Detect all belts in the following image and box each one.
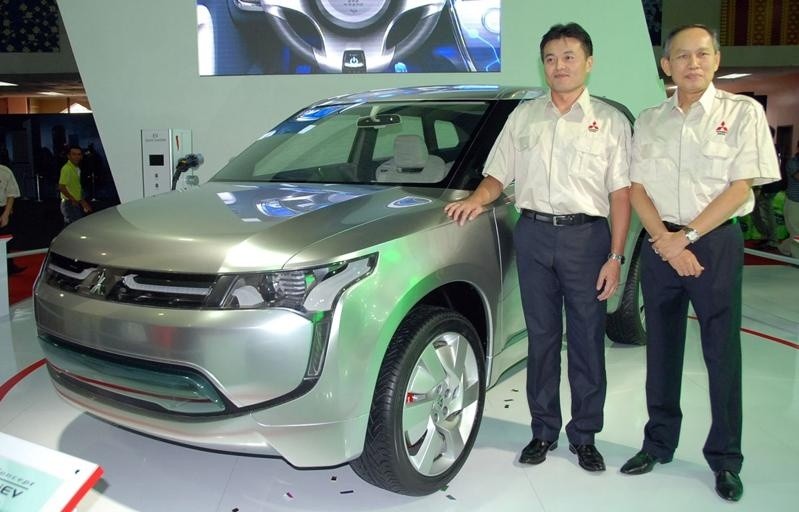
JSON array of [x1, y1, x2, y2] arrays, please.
[[663, 217, 737, 232], [521, 208, 602, 226]]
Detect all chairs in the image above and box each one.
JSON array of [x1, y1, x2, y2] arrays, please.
[[374, 134, 446, 184]]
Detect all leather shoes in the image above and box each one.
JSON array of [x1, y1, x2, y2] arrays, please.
[[569, 440, 605, 471], [620, 448, 673, 475], [519, 437, 558, 463], [715, 469, 743, 502]]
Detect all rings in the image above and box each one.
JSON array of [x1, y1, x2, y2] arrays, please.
[[654, 248, 660, 255]]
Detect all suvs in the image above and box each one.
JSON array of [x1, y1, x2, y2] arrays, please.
[[32, 84, 647, 498]]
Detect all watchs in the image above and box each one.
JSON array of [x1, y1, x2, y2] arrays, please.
[[684, 224, 701, 245], [606, 252, 627, 266]]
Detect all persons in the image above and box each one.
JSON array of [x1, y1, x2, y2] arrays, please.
[[56, 146, 92, 222], [1, 164, 29, 276], [443, 21, 633, 471], [619, 23, 782, 500], [739, 125, 798, 258]]
